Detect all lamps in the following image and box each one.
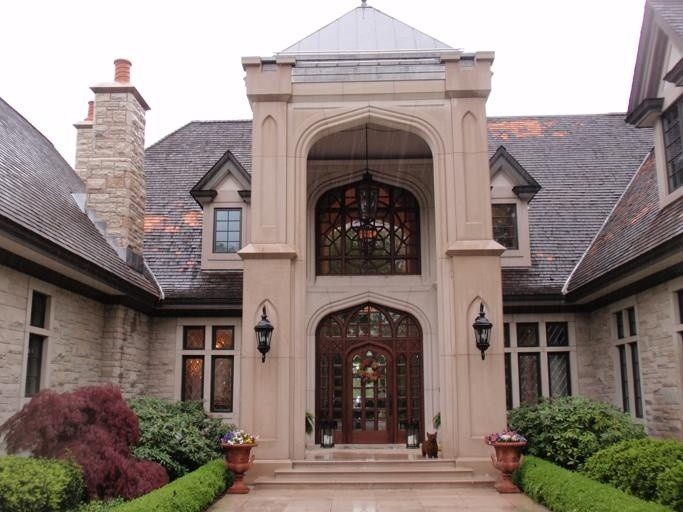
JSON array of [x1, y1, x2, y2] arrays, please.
[[254, 305, 274, 363], [472, 301, 492, 360], [355, 123, 380, 222]]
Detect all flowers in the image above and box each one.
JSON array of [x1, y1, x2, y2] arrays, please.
[[487, 430, 527, 442], [223, 429, 255, 444]]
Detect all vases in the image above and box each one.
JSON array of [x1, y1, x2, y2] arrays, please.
[[222, 444, 257, 494], [491, 442, 527, 493]]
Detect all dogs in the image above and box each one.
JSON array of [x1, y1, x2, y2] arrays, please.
[[421, 431, 439, 459]]
